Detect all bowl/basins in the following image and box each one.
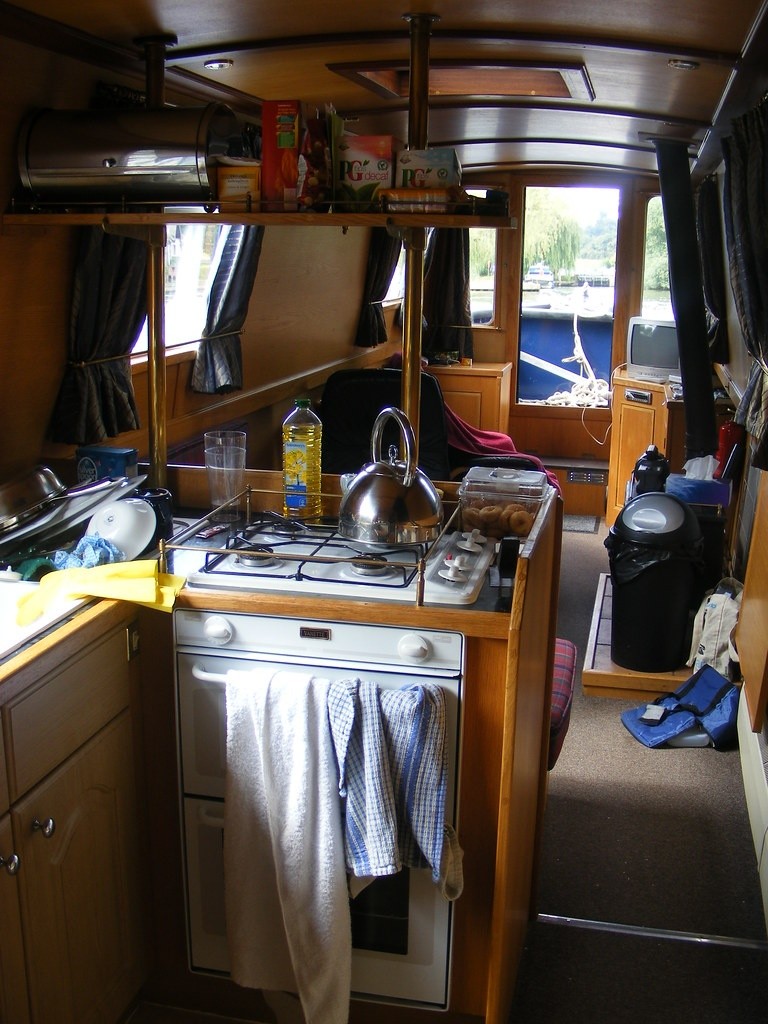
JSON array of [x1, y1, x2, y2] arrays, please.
[[436, 488, 444, 500]]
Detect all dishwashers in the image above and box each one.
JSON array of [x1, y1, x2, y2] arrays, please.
[[175, 609, 467, 1013]]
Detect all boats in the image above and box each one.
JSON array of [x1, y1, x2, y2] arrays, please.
[[522, 261, 554, 292]]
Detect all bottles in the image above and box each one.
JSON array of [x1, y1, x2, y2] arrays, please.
[[283, 398, 322, 525]]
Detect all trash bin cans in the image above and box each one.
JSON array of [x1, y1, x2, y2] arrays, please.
[[602, 491, 705, 672]]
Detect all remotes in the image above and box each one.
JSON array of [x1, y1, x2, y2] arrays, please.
[[635, 376, 665, 383]]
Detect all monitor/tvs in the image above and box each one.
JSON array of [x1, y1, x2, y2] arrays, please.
[[626, 317, 681, 380]]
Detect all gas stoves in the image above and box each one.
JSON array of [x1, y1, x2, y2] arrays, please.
[[187, 519, 500, 604]]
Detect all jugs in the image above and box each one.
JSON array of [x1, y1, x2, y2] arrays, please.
[[338, 407, 444, 544]]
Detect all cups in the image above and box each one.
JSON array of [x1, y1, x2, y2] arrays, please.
[[135, 488, 173, 542], [340, 474, 357, 494], [203, 430, 246, 522]]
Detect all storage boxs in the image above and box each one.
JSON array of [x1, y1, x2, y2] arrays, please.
[[332, 135, 461, 211], [456, 466, 551, 541], [217, 167, 262, 214], [76, 446, 139, 483]]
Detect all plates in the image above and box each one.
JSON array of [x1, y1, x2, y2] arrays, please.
[[85, 498, 156, 561]]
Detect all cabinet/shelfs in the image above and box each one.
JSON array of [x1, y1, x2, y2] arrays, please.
[[0, 598, 185, 1024]]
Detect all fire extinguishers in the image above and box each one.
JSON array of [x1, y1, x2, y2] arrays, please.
[[713, 407, 744, 480]]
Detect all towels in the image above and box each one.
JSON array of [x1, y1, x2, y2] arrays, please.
[[328, 677, 464, 901], [226, 670, 350, 1024]]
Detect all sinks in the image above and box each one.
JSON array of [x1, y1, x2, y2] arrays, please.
[[0, 581, 97, 662]]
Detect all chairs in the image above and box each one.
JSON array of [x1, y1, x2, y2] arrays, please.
[[322, 367, 537, 483]]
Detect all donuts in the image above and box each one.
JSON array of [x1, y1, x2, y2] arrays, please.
[[462, 499, 533, 535]]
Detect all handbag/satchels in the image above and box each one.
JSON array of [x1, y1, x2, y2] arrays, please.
[[620, 664, 740, 749]]
